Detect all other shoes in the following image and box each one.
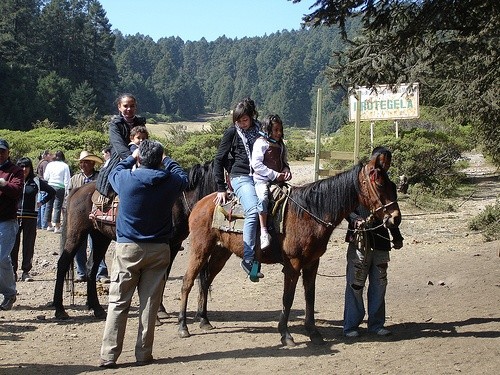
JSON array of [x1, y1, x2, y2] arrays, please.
[[47, 225, 62, 234], [346, 331, 359, 337], [100, 278, 110, 283], [242, 259, 264, 278], [73, 277, 86, 283], [38, 226, 47, 230], [137, 357, 154, 366], [376, 328, 392, 336], [97, 359, 117, 368]]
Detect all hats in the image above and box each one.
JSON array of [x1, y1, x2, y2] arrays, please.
[[0, 139, 9, 150], [72, 151, 103, 164]]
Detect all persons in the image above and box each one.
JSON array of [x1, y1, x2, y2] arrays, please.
[[343, 147, 393, 339], [127, 128, 155, 172], [98, 96, 145, 197], [251, 114, 291, 248], [0, 141, 110, 306], [98, 143, 189, 367], [213, 100, 268, 278]]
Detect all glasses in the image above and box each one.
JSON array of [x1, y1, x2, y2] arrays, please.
[[20, 165, 31, 168]]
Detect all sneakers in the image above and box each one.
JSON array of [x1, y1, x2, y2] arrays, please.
[[22, 273, 33, 282], [260, 233, 272, 249], [0, 295, 16, 310]]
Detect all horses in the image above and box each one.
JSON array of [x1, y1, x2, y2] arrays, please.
[[177, 152, 404, 348], [50, 156, 236, 320]]
